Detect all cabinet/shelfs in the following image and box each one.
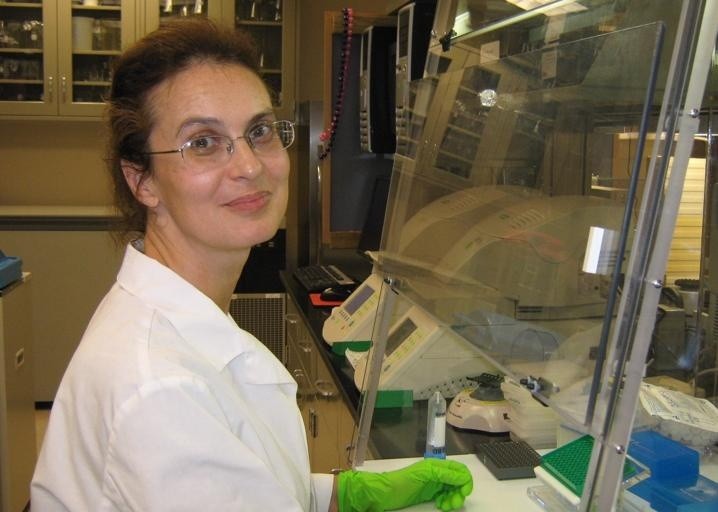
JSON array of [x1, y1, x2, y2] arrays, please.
[[283, 290, 374, 473], [141, 0, 295, 121], [0, 0, 141, 123]]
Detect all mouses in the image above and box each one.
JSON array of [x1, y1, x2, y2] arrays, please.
[[320, 287, 352, 301]]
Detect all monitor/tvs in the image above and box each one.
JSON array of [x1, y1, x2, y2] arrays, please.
[[357, 176, 391, 265]]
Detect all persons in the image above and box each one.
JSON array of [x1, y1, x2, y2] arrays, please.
[[30, 14, 473, 512]]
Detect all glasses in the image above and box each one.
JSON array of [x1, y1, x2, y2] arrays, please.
[[133, 118, 297, 170]]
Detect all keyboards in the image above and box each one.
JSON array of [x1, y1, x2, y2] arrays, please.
[[293, 264, 355, 292]]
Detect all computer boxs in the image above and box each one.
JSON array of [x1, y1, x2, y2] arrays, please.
[[360, 25, 396, 153], [396, 0, 438, 147]]
